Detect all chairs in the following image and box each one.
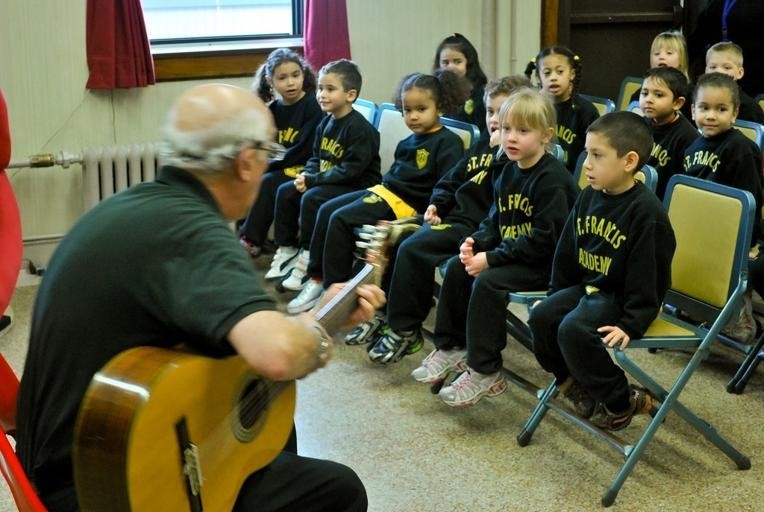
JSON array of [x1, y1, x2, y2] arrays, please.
[[0, 355, 53, 512], [734, 120, 760, 144], [352, 98, 659, 444], [517, 174, 760, 506], [575, 77, 646, 117]]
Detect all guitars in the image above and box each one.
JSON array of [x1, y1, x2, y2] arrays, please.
[[74, 225, 390, 511]]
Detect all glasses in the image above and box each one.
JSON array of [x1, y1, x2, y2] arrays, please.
[[258, 143, 286, 160]]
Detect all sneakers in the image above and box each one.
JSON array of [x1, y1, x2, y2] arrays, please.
[[345, 313, 423, 367], [264, 247, 324, 314], [411, 350, 507, 408], [560, 377, 654, 431], [722, 296, 757, 343]]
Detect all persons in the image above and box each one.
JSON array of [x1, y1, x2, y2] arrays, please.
[[411, 84, 582, 409], [527, 111, 676, 431], [525, 45, 600, 178], [234, 47, 328, 257], [433, 32, 488, 155], [286, 67, 473, 318], [263, 59, 382, 293], [15, 80, 368, 510], [344, 74, 534, 365]]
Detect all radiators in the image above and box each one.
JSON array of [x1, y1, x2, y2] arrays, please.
[[83, 139, 163, 215]]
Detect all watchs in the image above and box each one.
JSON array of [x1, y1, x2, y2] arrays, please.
[[313, 325, 331, 369]]
